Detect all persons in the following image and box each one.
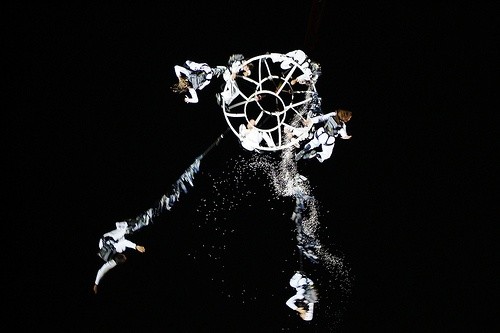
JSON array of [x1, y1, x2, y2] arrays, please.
[[236, 120, 268, 153], [297, 106, 356, 164], [267, 49, 323, 89], [286, 273, 319, 321], [213, 53, 254, 111], [90, 218, 147, 294], [172, 60, 226, 104]]
